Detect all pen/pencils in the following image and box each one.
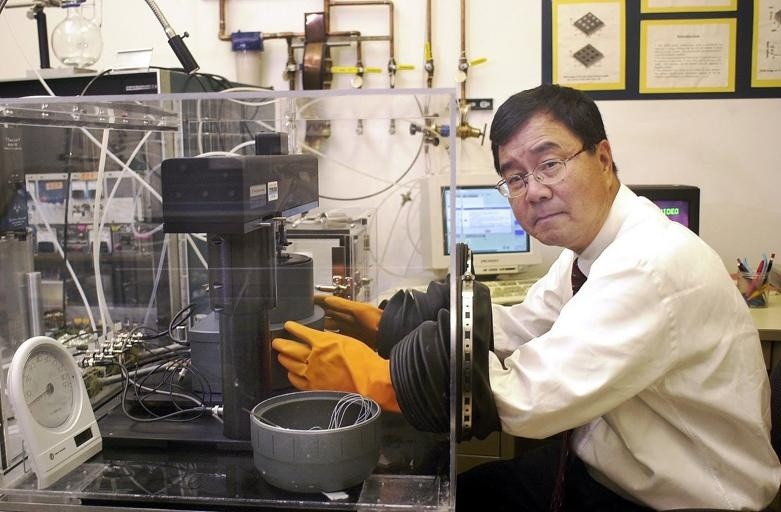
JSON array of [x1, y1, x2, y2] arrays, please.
[[736, 253, 775, 307]]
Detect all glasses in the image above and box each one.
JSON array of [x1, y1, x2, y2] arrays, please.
[[494, 143, 594, 199]]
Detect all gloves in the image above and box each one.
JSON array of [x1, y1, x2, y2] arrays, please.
[[271, 320, 404, 416], [313, 294, 384, 345]]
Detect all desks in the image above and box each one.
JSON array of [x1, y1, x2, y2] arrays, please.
[[457, 287, 781, 479]]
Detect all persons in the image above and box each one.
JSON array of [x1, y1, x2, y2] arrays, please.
[[272, 85, 781, 511]]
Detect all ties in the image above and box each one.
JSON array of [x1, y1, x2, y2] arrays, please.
[[571, 256, 587, 296]]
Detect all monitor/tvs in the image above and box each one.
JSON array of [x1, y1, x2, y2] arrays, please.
[[625, 184, 700, 237], [429, 174, 542, 274]]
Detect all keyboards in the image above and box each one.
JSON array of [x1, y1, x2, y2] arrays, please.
[[480, 279, 539, 306]]
